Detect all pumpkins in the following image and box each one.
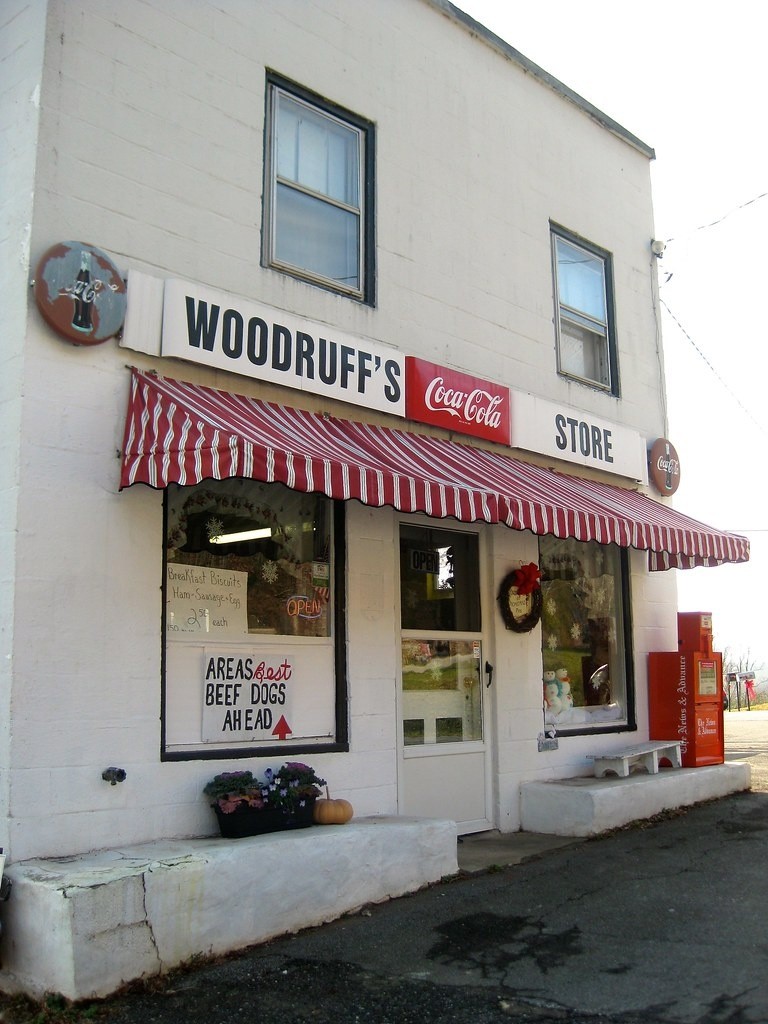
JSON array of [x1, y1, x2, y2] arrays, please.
[[313, 786, 353, 824]]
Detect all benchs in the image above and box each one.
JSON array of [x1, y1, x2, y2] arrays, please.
[[585, 739, 685, 777]]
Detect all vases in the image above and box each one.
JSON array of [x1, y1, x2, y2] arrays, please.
[[207, 794, 320, 839]]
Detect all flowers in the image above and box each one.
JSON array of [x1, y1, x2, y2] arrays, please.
[[200, 770, 264, 814], [262, 760, 328, 814]]
